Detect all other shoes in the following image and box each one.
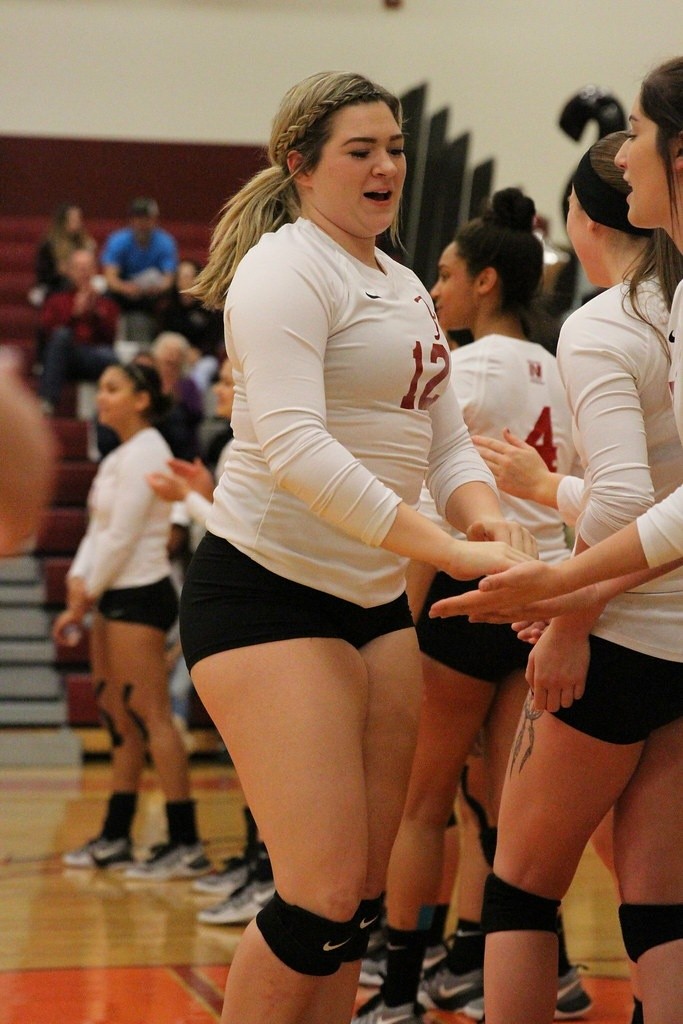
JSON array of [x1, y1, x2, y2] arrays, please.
[[353, 922, 591, 1023], [64, 832, 274, 923]]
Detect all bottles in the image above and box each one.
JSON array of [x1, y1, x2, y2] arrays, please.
[[53, 617, 82, 646]]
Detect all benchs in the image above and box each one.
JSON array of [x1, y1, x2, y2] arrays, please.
[[1, 214, 229, 729]]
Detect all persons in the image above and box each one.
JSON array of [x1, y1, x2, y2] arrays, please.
[[172, 70, 539, 1024], [351, 57, 683, 1024], [1, 200, 276, 923]]
